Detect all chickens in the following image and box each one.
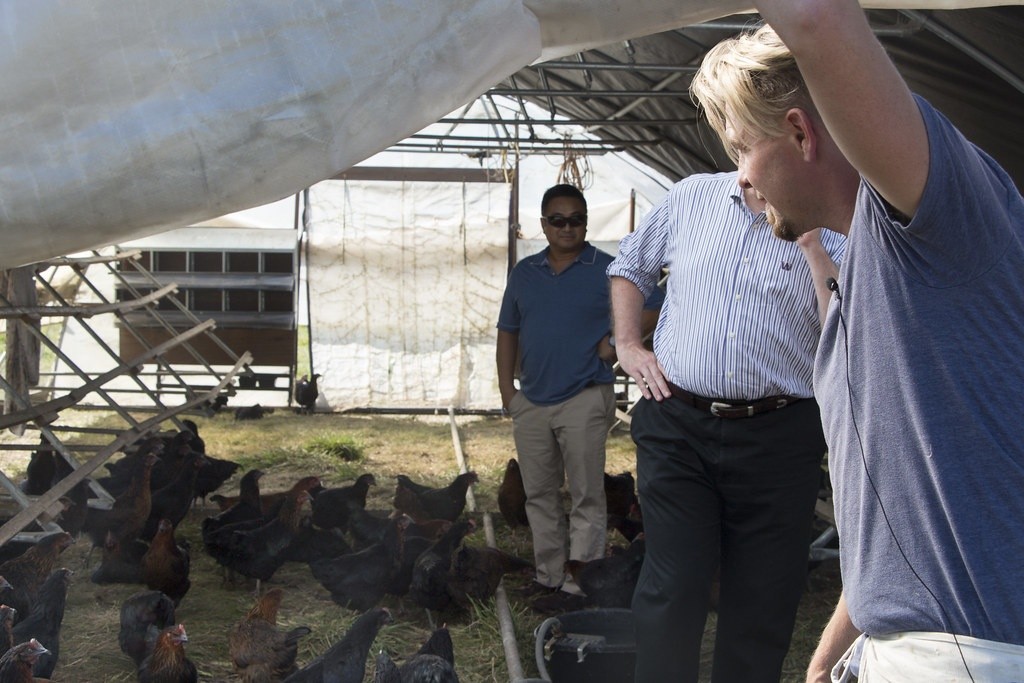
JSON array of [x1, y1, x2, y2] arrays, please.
[[607, 509, 642, 541], [118, 589, 176, 669], [136, 622, 198, 683], [278, 606, 395, 683], [396, 622, 459, 683], [18, 418, 549, 629], [561, 549, 642, 608], [615, 392, 629, 421], [0, 497, 78, 566], [604, 471, 638, 517], [0, 531, 78, 683], [374, 648, 402, 683], [227, 587, 312, 683], [295, 373, 322, 415]]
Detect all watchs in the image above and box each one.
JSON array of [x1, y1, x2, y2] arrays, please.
[[607, 332, 615, 349]]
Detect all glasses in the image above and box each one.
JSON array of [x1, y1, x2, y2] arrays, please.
[[543, 214, 587, 228]]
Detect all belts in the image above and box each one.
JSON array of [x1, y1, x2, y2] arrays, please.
[[664, 381, 800, 419]]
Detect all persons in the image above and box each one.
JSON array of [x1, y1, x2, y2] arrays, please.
[[688, 1, 1023, 683], [604, 102, 850, 683], [496, 184, 668, 618]]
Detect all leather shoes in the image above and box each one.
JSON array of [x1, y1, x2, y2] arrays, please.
[[513, 581, 586, 615]]
